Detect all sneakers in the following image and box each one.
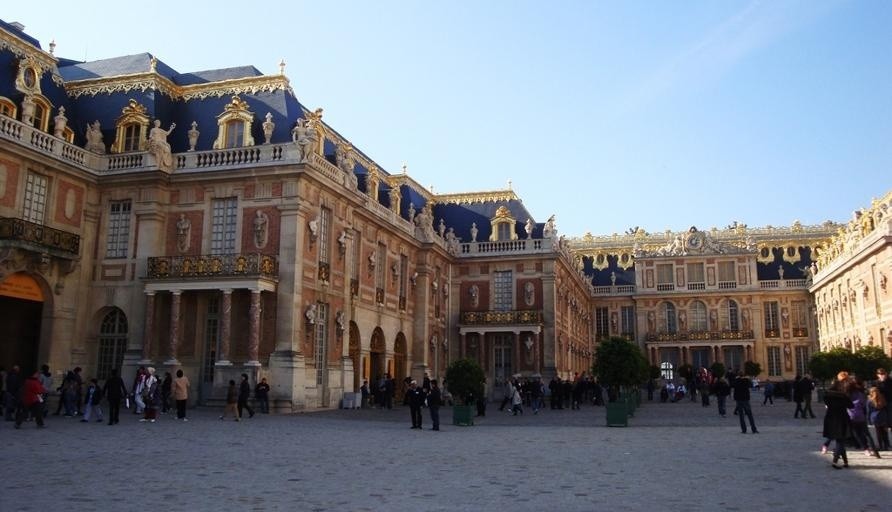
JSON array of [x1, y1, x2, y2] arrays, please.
[[832, 464, 850, 470], [740, 431, 746, 434], [821, 445, 828, 455], [864, 450, 873, 457], [752, 430, 760, 434], [248, 411, 255, 418], [138, 418, 156, 423]]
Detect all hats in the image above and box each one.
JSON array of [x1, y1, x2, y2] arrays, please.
[[147, 367, 156, 376]]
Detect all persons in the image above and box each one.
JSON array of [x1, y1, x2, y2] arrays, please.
[[220, 380, 240, 422], [0, 363, 190, 430], [292, 118, 316, 164], [237, 373, 255, 419], [256, 377, 270, 414], [657, 365, 775, 434], [800, 371, 816, 419], [793, 375, 806, 419], [414, 208, 432, 239], [819, 367, 892, 470], [360, 373, 454, 431], [497, 370, 606, 417], [147, 120, 176, 167]]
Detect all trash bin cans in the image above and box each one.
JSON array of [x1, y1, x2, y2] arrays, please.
[[343, 392, 362, 410]]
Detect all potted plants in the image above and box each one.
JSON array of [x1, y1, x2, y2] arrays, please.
[[444, 359, 487, 427], [809, 348, 831, 404], [593, 333, 648, 425]]
